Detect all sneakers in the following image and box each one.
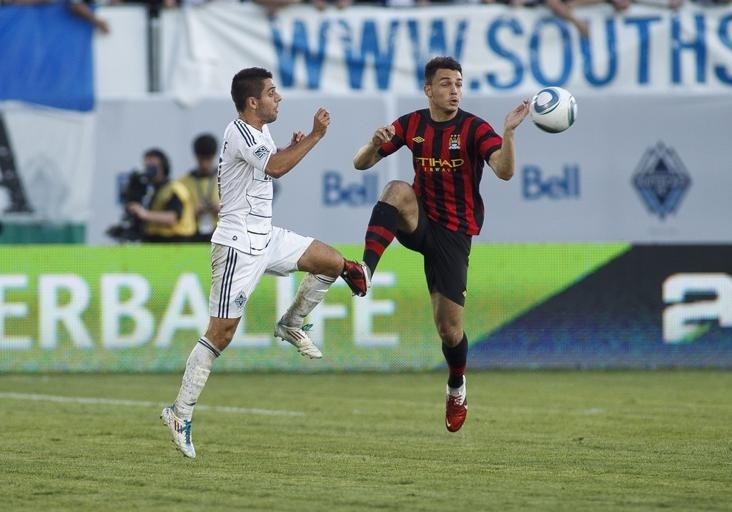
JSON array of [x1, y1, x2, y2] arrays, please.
[[445, 375, 468, 432], [340, 257, 371, 297], [273, 320, 323, 358], [160, 406, 197, 458]]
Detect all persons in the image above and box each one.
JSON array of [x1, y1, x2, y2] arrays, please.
[[160, 66, 349, 460], [123, 148, 199, 241], [179, 134, 220, 242], [338, 57, 532, 432], [67, 0, 686, 41]]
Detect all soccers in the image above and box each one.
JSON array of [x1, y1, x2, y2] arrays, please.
[[531, 87, 576, 133]]
